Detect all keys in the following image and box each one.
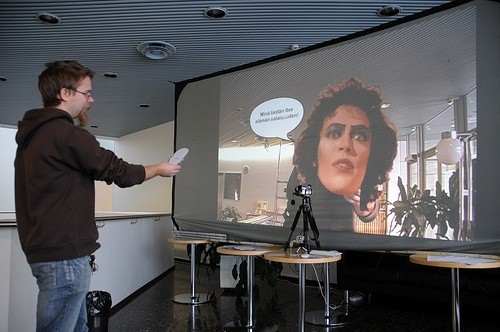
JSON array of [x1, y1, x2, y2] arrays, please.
[[88, 254, 98, 273]]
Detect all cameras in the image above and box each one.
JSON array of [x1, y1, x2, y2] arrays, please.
[[292, 185, 313, 196]]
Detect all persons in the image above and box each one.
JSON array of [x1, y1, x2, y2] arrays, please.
[[282, 78, 398, 231], [14, 60, 181, 332]]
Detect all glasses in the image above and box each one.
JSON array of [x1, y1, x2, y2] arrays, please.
[[65, 87, 92, 97]]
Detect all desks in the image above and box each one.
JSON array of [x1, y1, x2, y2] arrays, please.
[[167, 238, 354, 332], [410, 254, 500, 332]]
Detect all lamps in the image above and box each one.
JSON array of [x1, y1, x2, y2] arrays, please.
[[435, 132, 478, 166]]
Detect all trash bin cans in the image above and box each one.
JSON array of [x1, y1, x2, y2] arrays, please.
[[85, 288, 113, 330]]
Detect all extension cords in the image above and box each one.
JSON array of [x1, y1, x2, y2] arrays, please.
[[350, 296, 363, 302]]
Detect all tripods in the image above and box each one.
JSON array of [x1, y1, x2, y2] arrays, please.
[[284, 196, 321, 254]]
